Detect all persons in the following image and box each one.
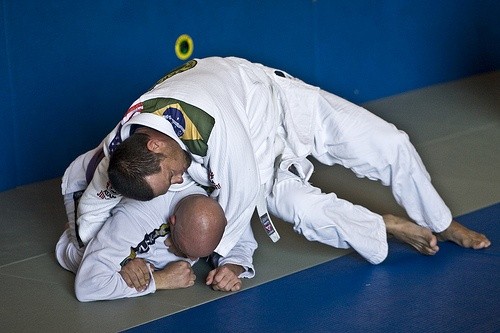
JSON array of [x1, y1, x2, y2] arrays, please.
[[75, 55, 492, 292], [54, 143, 260, 303]]
